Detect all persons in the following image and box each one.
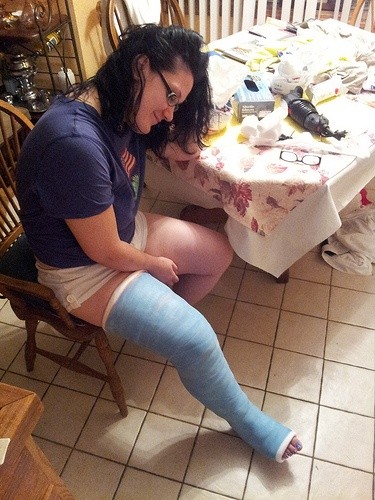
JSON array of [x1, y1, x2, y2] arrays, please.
[[16, 23, 302, 464]]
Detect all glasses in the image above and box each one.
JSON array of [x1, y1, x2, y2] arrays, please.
[[279, 130, 322, 166], [150, 62, 179, 112]]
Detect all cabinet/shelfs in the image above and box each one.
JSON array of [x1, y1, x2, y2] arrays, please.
[[0, 0, 85, 125]]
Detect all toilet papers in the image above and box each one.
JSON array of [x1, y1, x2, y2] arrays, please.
[[256, 98, 289, 134], [262, 120, 287, 143], [240, 115, 259, 137]]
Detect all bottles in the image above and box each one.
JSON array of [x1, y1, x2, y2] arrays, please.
[[21, 0, 43, 31], [58, 67, 79, 98], [31, 28, 64, 61]]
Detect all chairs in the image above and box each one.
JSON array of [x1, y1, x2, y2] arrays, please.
[[0, 99, 131, 417], [106, 0, 186, 52]]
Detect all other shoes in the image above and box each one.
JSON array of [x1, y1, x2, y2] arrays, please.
[[179, 204, 228, 223]]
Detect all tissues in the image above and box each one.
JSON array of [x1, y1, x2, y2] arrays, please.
[[271, 59, 310, 96], [230, 74, 275, 123]]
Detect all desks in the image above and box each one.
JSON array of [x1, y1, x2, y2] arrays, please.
[[146, 16, 375, 283], [0, 383, 76, 500]]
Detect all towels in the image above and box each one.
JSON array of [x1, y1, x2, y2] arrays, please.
[[320, 202, 375, 276], [108, 0, 162, 39]]
